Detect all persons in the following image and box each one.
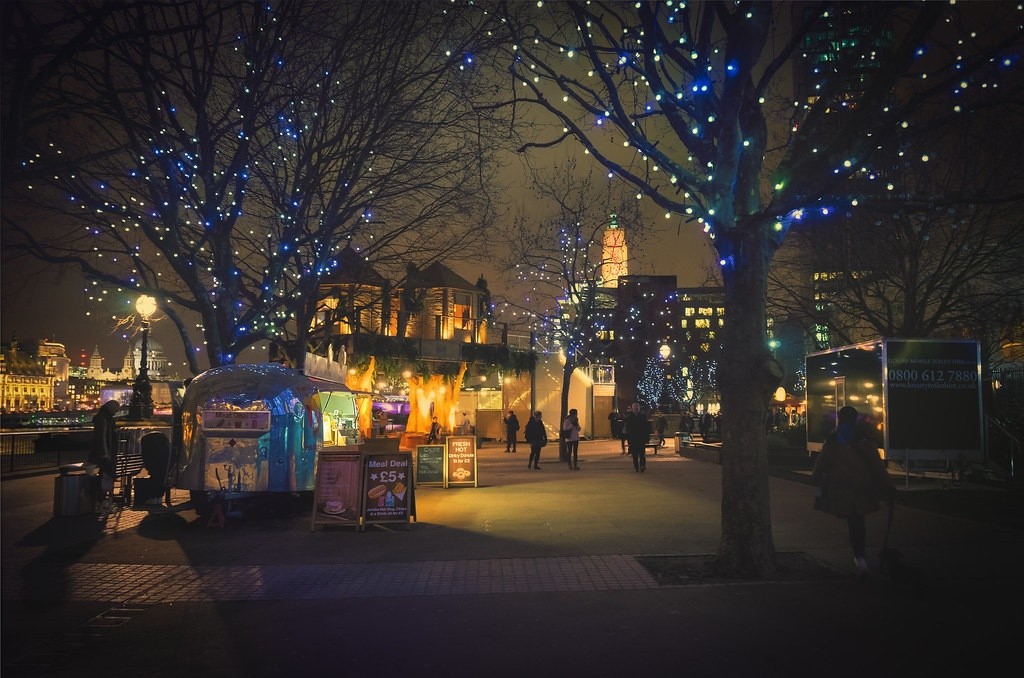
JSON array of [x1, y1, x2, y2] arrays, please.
[[716, 411, 721, 435], [810, 406, 896, 580], [428, 417, 439, 444], [621, 403, 650, 473], [679, 412, 694, 439], [503, 411, 519, 453], [698, 409, 711, 438], [655, 413, 668, 446], [563, 409, 581, 470], [524, 411, 547, 470], [456, 413, 470, 436], [608, 408, 618, 440], [89, 400, 120, 514]]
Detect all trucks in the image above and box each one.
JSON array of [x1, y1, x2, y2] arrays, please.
[[169, 363, 386, 512]]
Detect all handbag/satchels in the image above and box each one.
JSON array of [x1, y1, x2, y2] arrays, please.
[[560, 430, 571, 438]]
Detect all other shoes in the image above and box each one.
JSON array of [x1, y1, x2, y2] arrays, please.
[[512, 449, 516, 452], [640, 467, 645, 472], [855, 558, 867, 569], [534, 464, 541, 469], [504, 450, 510, 453], [574, 466, 580, 471], [635, 466, 639, 471], [568, 465, 573, 472], [528, 465, 531, 471]]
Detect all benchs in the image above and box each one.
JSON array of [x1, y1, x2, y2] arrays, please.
[[59, 453, 144, 503], [623, 433, 660, 455]]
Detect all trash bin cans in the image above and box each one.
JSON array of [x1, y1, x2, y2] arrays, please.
[[674, 432, 690, 453], [53, 462, 100, 522]]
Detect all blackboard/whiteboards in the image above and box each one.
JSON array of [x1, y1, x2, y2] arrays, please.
[[446, 436, 478, 484], [361, 451, 417, 524], [415, 444, 446, 484], [310, 451, 364, 525]]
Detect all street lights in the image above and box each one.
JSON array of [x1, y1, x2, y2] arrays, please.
[[129, 294, 156, 418]]
